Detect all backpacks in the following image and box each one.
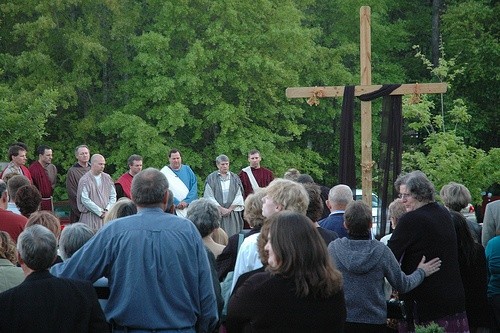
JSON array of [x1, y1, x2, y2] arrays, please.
[[481, 181, 500, 219]]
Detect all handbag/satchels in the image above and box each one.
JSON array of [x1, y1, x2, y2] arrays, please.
[[219, 233, 244, 317]]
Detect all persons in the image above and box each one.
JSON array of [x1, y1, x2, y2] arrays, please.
[[76, 154, 117, 230], [204, 155, 246, 240], [66, 145, 93, 218], [49, 167, 219, 333], [238, 150, 274, 198], [114, 154, 144, 200], [0, 143, 33, 184], [0, 167, 500, 333], [160, 149, 197, 219], [29, 145, 57, 211]]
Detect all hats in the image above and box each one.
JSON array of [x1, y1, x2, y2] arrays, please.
[[297, 174, 314, 184]]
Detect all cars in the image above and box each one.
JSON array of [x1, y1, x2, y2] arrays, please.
[[53, 200, 71, 231], [352, 188, 390, 237]]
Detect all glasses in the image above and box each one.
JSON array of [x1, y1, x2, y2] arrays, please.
[[397, 193, 409, 198]]
